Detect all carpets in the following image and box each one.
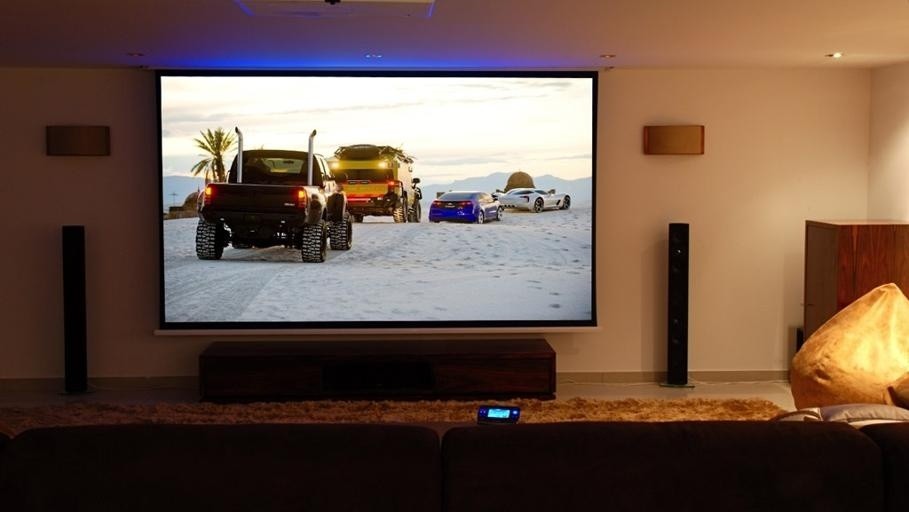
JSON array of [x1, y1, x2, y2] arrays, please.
[[0, 398, 797, 438]]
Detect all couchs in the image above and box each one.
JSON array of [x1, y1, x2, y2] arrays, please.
[[0, 420, 909, 512]]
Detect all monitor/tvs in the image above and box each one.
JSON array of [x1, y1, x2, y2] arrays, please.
[[153, 69, 602, 338]]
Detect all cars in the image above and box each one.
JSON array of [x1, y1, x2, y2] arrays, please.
[[491, 187, 571, 213]]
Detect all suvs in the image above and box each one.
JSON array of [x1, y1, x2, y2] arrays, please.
[[324, 144, 422, 223], [195, 126, 353, 263]]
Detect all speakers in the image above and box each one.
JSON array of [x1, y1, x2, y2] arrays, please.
[[57, 225, 97, 394], [659, 222, 695, 388]]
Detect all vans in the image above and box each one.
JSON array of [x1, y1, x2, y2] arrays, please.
[[429, 191, 504, 224]]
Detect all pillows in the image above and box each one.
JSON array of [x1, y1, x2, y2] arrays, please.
[[791, 283, 909, 410]]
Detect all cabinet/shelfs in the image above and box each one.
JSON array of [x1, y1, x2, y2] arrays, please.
[[803, 218, 909, 343], [197, 340, 556, 403]]
